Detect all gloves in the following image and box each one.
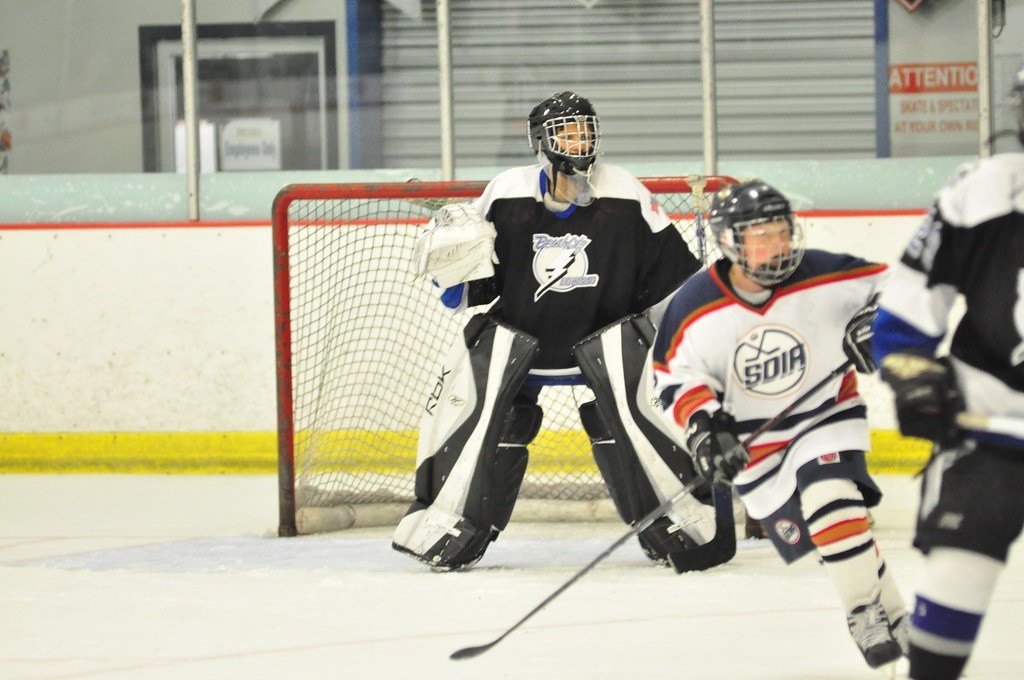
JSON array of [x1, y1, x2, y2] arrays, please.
[[843, 293, 882, 373], [883, 358, 971, 448], [685, 407, 751, 480]]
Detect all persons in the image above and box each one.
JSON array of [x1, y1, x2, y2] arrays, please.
[[644, 179, 910, 669], [872, 70, 1024, 680], [386, 93, 736, 574]]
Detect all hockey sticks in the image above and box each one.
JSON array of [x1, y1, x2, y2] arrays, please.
[[448, 355, 856, 662]]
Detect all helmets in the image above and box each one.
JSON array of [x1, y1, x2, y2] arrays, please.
[[710, 180, 806, 286], [527, 92, 601, 176]]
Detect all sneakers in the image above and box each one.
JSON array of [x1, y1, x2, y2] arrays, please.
[[847, 591, 911, 667]]
